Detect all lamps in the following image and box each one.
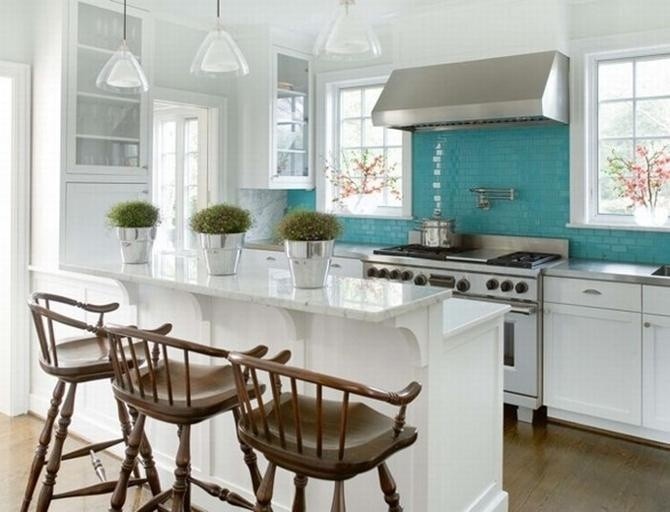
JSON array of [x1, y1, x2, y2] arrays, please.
[[311, 0, 382, 66], [192, 1, 250, 80], [94, 0, 150, 97]]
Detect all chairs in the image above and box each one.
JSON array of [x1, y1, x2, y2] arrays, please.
[[98, 323, 266, 511], [223, 352, 422, 511], [20, 293, 162, 511]]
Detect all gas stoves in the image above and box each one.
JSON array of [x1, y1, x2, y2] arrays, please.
[[373, 243, 561, 269]]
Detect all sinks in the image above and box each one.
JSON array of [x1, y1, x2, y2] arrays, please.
[[650, 265, 670, 278]]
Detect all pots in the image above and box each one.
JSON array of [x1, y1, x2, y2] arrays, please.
[[407, 208, 456, 249]]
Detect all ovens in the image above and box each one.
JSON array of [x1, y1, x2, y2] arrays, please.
[[492, 295, 545, 411]]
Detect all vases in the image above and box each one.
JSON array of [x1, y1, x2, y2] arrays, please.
[[344, 194, 377, 213], [634, 206, 668, 226]]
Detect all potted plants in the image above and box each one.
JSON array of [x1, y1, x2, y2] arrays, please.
[[189, 203, 254, 277], [103, 201, 162, 264], [276, 212, 341, 289]]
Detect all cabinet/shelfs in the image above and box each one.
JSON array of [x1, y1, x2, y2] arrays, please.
[[642, 282, 670, 445], [240, 40, 315, 192], [32, 0, 152, 267], [543, 269, 642, 439], [244, 245, 362, 280]]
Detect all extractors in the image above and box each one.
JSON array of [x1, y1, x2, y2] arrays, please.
[[371, 51, 570, 132]]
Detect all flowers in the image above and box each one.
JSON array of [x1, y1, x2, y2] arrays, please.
[[319, 146, 402, 205], [605, 139, 670, 210]]
